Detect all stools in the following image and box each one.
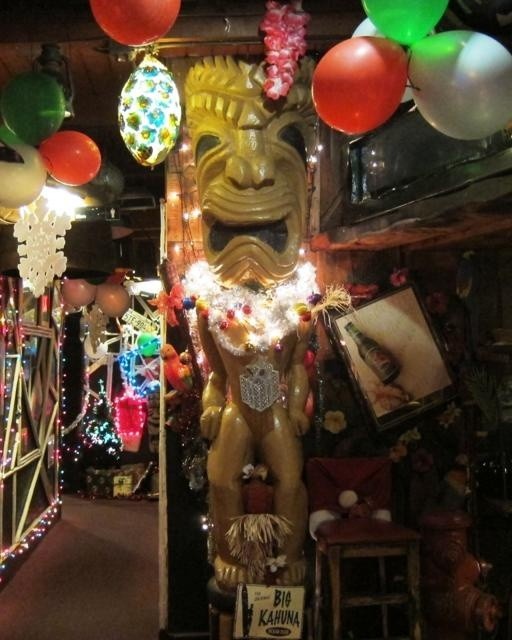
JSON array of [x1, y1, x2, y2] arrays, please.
[[314, 516, 425, 636]]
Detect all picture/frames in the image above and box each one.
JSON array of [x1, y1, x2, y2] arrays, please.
[[332, 280, 464, 434]]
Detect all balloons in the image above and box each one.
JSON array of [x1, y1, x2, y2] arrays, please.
[[62, 278, 131, 318], [0, 143, 47, 207], [90, 0, 181, 46], [137, 334, 160, 358], [0, 71, 65, 147], [38, 131, 102, 186], [311, 0, 512, 140]]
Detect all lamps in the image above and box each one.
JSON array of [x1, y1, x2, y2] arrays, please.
[[116, 53, 182, 169]]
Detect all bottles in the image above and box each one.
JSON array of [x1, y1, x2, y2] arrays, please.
[[342, 321, 402, 385]]
